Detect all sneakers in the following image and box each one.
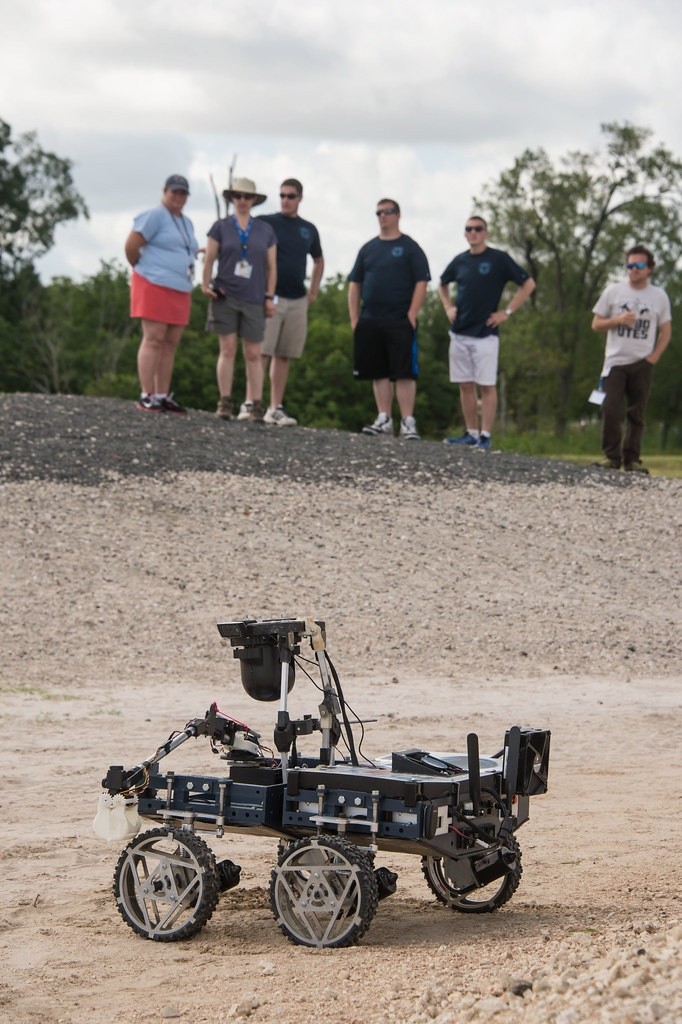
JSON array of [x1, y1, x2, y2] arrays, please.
[[135, 395, 165, 413], [399, 416, 420, 439], [362, 413, 393, 435], [473, 435, 491, 452], [444, 432, 479, 446], [153, 393, 187, 415], [262, 408, 297, 427], [237, 405, 263, 420]]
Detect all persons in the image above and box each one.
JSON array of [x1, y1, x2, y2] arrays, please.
[[124, 175, 197, 414], [348, 199, 432, 439], [195, 176, 324, 428], [591, 245, 672, 474], [436, 216, 537, 449]]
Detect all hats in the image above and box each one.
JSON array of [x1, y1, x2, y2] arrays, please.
[[222, 178, 266, 206], [166, 175, 189, 194]]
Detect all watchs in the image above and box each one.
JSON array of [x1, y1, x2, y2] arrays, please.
[[505, 308, 512, 315]]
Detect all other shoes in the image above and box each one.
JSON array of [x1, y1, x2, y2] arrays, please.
[[592, 459, 620, 469], [216, 401, 234, 419], [625, 459, 649, 474]]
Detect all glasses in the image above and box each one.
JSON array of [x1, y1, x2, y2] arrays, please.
[[466, 226, 486, 232], [233, 193, 251, 200], [280, 192, 300, 199], [627, 262, 649, 269], [377, 208, 397, 215]]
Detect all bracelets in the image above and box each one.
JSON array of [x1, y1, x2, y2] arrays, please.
[[265, 292, 275, 299]]
